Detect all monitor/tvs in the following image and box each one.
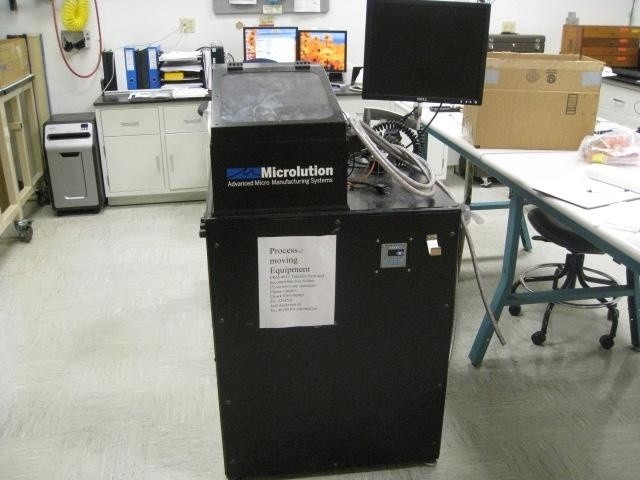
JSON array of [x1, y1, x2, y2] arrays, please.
[[242, 26, 298, 70], [299, 30, 349, 89], [361, 0, 491, 106]]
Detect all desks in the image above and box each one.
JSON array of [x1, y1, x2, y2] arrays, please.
[[393, 94, 639, 368]]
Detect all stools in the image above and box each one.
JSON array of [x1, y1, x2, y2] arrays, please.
[[509, 203, 622, 353]]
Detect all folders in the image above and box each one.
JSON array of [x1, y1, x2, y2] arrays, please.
[[147, 44, 161, 89], [124, 48, 138, 90]]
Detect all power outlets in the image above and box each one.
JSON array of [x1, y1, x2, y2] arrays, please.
[[180, 17, 195, 32]]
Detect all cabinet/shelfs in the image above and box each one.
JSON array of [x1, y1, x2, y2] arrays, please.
[[98, 102, 211, 197], [599, 81, 639, 130], [333, 93, 449, 179]]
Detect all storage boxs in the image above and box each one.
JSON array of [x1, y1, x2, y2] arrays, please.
[[461, 47, 602, 153]]
